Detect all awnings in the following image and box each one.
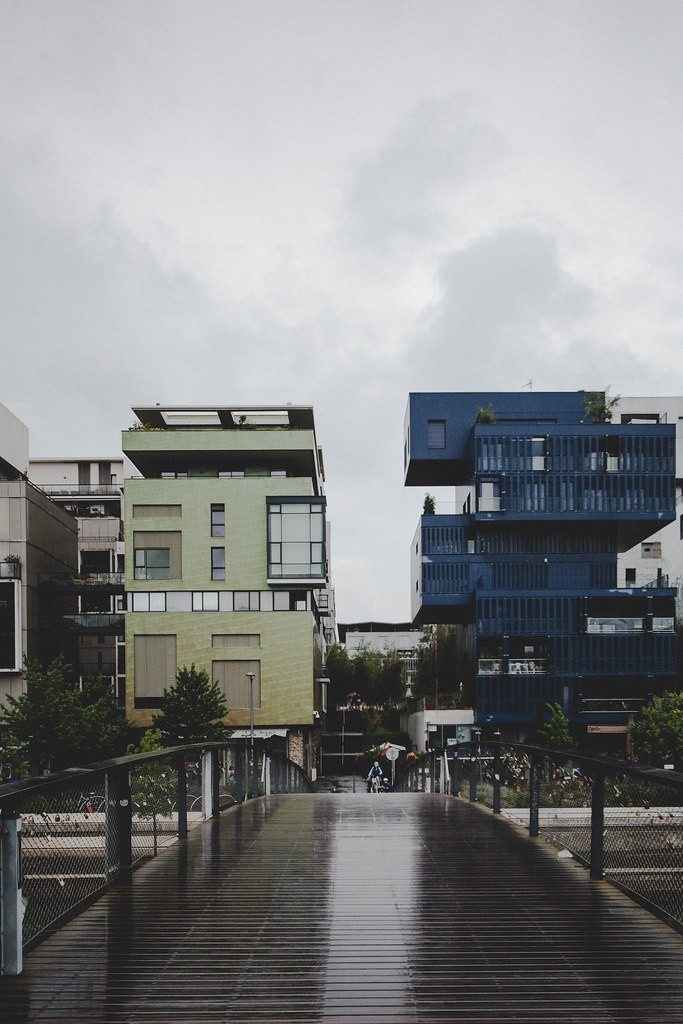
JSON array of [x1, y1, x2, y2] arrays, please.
[[157, 728, 290, 740]]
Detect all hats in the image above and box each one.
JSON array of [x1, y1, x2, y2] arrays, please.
[[374, 762, 379, 767], [383, 778, 388, 782]]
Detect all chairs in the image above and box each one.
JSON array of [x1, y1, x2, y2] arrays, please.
[[492, 662, 539, 673]]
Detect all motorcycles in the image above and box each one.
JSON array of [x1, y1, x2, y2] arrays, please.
[[367, 773, 390, 793]]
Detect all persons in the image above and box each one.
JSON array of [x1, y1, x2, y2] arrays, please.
[[227, 766, 236, 787], [366, 761, 383, 787], [492, 660, 537, 674], [381, 777, 392, 793]]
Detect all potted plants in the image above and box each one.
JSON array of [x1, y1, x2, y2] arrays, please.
[[4, 555, 20, 576]]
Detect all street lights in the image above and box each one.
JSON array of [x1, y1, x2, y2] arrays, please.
[[246, 669, 256, 763]]
